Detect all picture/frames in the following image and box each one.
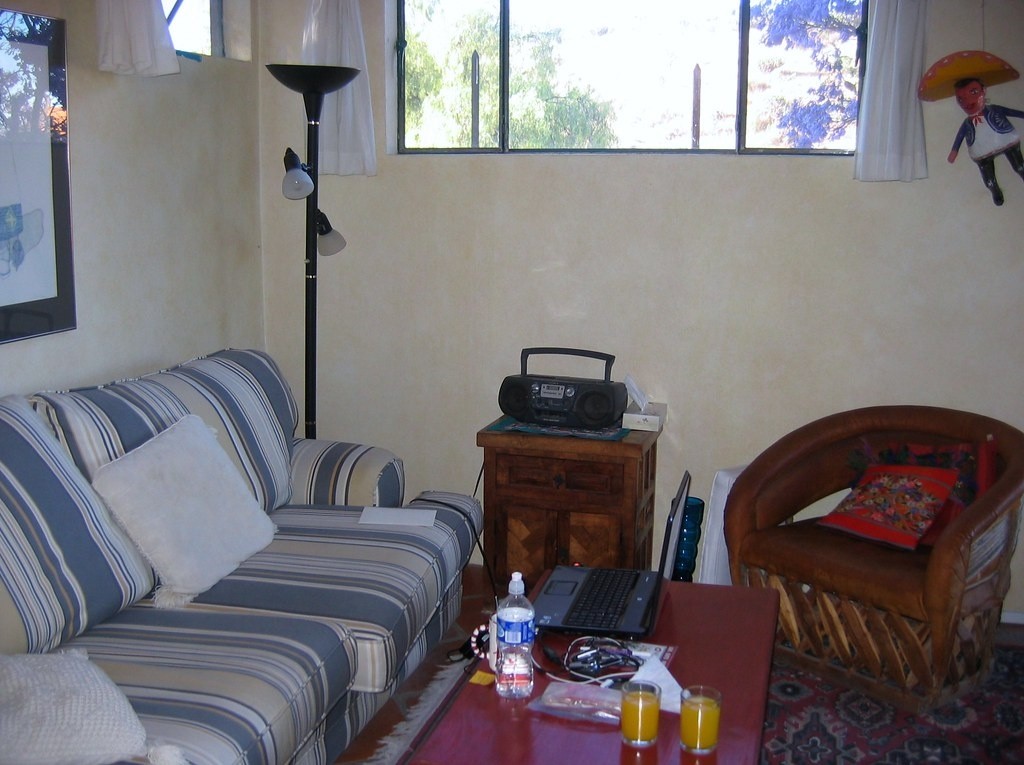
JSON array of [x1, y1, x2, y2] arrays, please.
[[0, 9, 78, 344]]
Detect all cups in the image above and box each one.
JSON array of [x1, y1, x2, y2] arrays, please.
[[679, 684, 723, 755], [620, 680, 662, 748], [489, 608, 533, 674]]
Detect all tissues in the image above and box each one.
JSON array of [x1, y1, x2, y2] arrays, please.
[[622, 374, 668, 432]]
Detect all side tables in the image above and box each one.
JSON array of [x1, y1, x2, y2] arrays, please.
[[478, 402, 663, 598]]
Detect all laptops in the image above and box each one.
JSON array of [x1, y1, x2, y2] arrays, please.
[[531, 471, 691, 639]]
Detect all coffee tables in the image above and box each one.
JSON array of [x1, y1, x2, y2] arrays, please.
[[404, 568, 780, 765]]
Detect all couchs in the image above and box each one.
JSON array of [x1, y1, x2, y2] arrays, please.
[[0, 346, 484, 765], [722, 405, 1024, 714]]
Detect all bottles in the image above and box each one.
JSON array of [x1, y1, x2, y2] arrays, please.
[[496, 571, 535, 698]]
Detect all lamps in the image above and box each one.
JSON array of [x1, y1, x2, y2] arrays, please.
[[282, 147, 315, 201], [317, 208, 346, 257]]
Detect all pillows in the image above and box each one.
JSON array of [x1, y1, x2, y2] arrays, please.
[[91, 415, 280, 608], [815, 439, 998, 550], [0, 647, 192, 765]]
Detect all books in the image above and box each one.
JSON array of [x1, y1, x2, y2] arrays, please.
[[358, 506, 437, 526]]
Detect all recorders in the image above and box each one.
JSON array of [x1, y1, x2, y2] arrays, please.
[[498, 348, 629, 431]]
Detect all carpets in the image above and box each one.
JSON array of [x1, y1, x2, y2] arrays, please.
[[360, 629, 1023, 765]]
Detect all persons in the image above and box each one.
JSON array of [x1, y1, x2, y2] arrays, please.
[[947, 77, 1024, 206]]
